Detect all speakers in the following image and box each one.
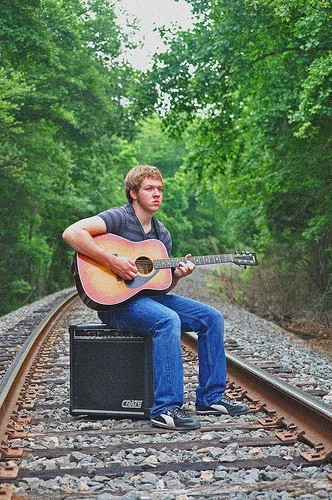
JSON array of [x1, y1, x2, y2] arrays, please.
[[68, 322, 155, 420]]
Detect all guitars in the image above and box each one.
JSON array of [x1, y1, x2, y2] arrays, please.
[[71, 231, 259, 311]]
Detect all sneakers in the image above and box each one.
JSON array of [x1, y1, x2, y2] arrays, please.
[[194, 395, 252, 416], [148, 408, 201, 430]]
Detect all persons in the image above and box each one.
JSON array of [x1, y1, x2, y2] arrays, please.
[[63, 164, 249, 430]]
